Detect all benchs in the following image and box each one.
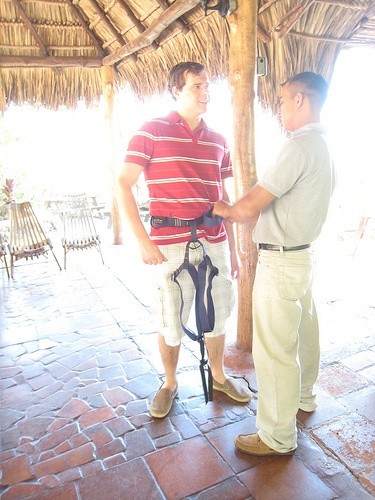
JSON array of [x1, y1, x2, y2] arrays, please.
[[54, 206, 105, 221]]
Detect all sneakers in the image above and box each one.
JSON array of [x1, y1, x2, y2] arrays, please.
[[149, 381, 179, 418], [234, 433, 296, 456], [212, 376, 253, 403]]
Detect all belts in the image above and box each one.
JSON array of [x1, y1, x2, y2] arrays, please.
[[258, 243, 312, 251]]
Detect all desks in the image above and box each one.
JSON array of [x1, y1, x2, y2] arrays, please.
[[44, 195, 104, 221]]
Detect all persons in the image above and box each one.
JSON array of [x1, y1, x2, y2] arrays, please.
[[114, 63, 252, 418], [212, 72, 335, 455]]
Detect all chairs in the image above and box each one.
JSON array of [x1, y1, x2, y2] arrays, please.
[[0, 192, 105, 278]]
